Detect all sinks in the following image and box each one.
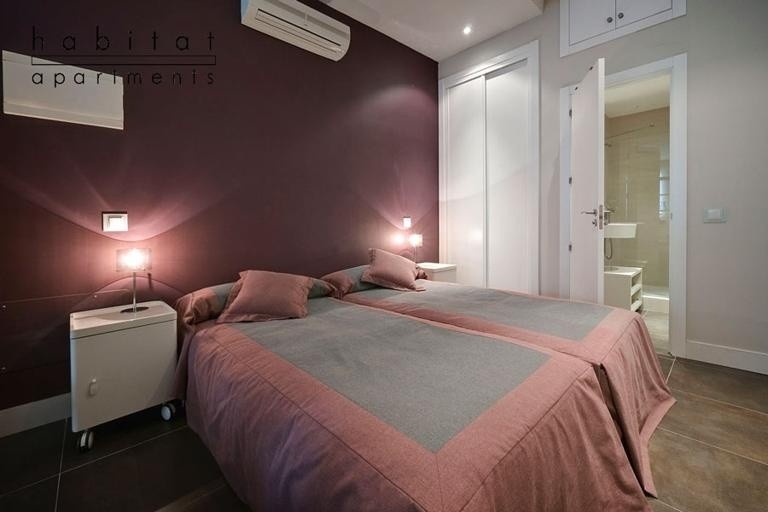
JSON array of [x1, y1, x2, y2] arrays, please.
[[603, 222, 645, 239]]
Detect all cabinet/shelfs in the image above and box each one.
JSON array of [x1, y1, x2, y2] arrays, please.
[[603, 262, 644, 314], [434, 40, 541, 300], [558, 0, 688, 57]]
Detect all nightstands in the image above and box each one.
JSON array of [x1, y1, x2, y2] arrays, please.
[[414, 261, 457, 284], [64, 299, 181, 452]]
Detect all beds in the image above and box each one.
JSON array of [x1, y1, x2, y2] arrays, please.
[[169, 273, 650, 512], [318, 261, 678, 502]]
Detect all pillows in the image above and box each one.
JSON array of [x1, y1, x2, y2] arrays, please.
[[360, 246, 428, 293], [172, 274, 336, 325], [216, 267, 311, 324], [322, 263, 428, 297]]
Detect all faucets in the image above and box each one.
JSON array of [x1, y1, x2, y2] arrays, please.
[[605, 210, 613, 225]]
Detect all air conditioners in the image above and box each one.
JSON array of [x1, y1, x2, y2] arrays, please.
[[238, 0, 351, 62]]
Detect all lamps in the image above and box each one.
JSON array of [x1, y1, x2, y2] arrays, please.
[[409, 231, 423, 264], [115, 245, 151, 316]]
